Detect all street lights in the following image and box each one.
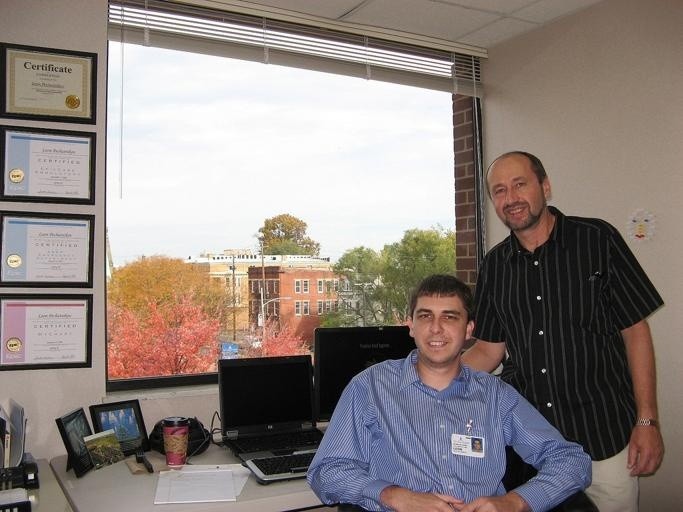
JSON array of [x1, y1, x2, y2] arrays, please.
[[260, 286, 293, 357]]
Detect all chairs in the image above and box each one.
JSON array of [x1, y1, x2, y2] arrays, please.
[[498, 441, 600, 511]]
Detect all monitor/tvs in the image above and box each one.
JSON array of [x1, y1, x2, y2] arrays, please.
[[313, 325, 417, 421]]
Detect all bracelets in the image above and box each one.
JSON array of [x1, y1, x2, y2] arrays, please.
[[634, 417, 660, 428]]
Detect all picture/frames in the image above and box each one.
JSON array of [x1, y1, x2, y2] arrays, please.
[[1, 123, 98, 207], [55, 407, 94, 480], [0, 291, 95, 372], [0, 209, 96, 291], [88, 397, 155, 456], [0, 42, 99, 125]]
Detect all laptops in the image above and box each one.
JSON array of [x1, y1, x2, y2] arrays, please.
[[217, 354, 324, 462]]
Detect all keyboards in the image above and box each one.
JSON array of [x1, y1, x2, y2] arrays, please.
[[246, 453, 317, 482]]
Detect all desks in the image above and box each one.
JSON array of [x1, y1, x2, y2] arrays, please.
[[49, 438, 342, 511], [0, 454, 76, 512]]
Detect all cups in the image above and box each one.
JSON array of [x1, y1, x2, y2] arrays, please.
[[160, 416, 190, 466]]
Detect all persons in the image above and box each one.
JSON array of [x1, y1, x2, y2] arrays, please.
[[307, 273, 591, 512], [472, 437, 483, 453], [458, 152, 663, 512]]
